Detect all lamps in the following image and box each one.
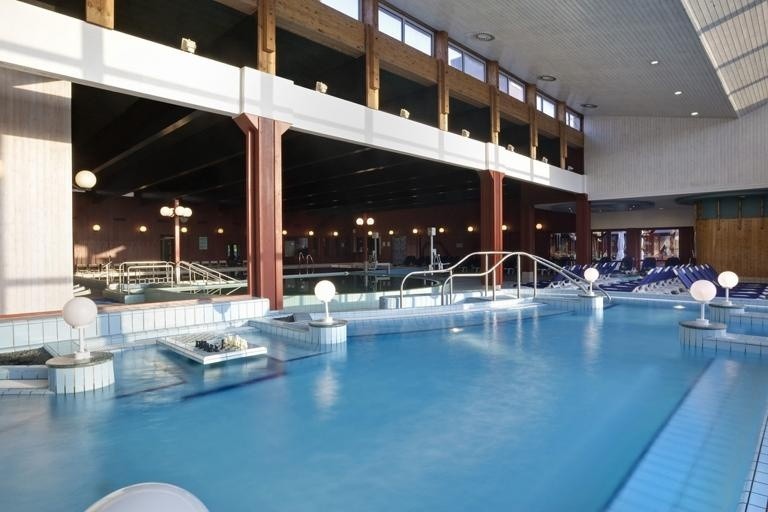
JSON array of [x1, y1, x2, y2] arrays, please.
[[718, 271, 740, 306], [584, 267, 599, 297], [62, 297, 98, 363], [690, 279, 717, 324], [315, 279, 336, 325]]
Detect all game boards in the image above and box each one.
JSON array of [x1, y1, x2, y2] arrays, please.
[[190, 338, 258, 355], [170, 333, 220, 345]]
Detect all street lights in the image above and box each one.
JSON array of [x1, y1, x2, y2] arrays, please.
[[160, 206, 192, 284], [356, 217, 374, 286]]
